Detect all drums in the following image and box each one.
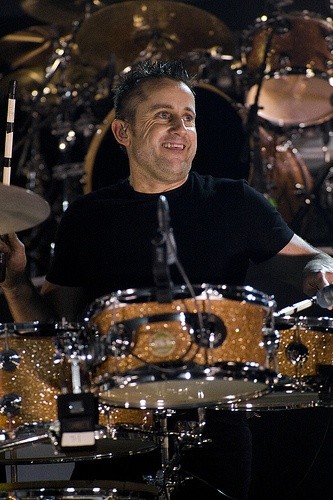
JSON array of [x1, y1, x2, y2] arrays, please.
[[0, 320, 161, 464], [81, 79, 314, 234], [221, 313, 332, 410], [236, 13, 332, 127], [86, 283, 278, 411]]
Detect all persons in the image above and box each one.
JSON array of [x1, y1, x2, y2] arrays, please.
[[0, 58, 333, 500]]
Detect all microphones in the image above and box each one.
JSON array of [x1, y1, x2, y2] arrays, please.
[[154, 196, 177, 265]]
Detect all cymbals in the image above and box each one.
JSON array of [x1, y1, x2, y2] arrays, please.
[[19, 0, 85, 27], [0, 182, 52, 236], [1, 64, 110, 100], [75, 1, 236, 69], [0, 22, 109, 68]]
